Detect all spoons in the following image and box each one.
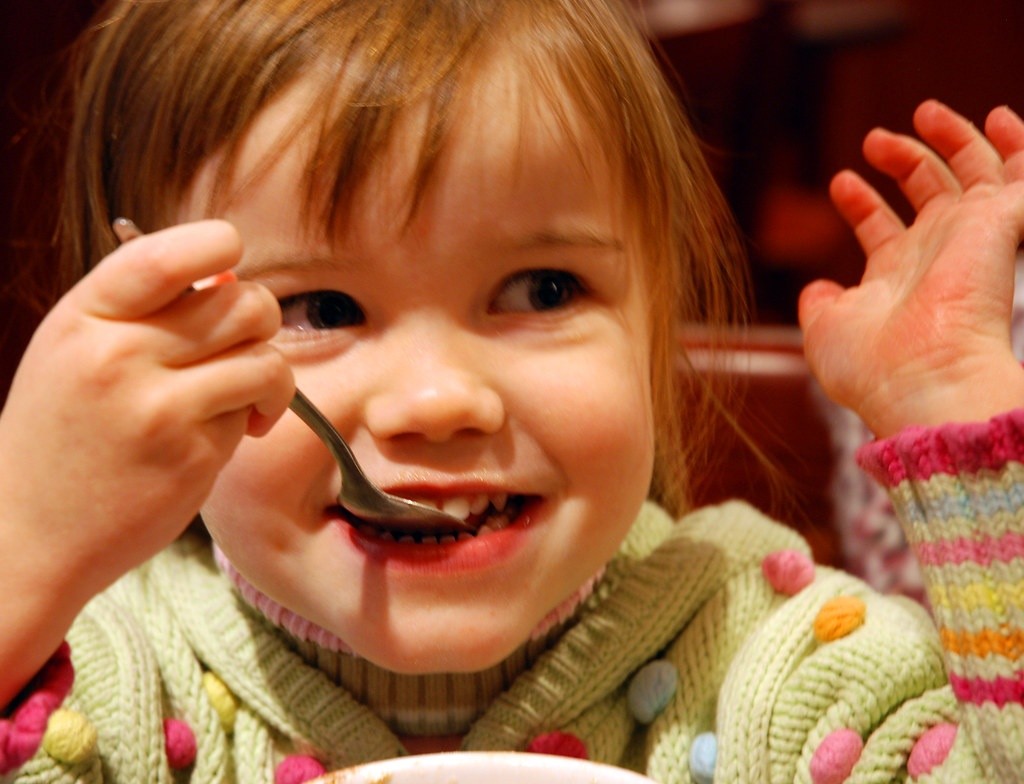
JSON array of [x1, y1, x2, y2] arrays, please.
[[113, 219, 480, 535]]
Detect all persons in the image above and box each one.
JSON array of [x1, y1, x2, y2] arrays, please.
[[0, 0, 1024, 784]]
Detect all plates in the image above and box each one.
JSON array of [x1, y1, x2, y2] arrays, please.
[[294, 751, 660, 784]]
